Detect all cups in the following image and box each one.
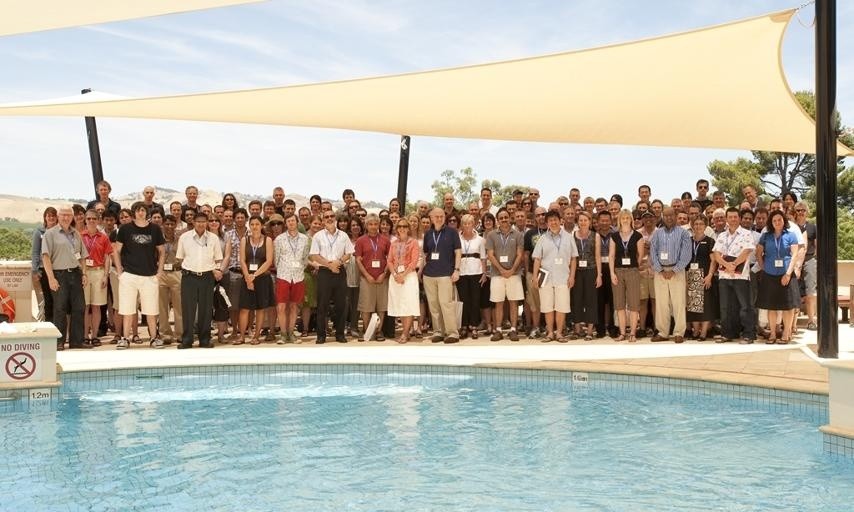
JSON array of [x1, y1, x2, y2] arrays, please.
[[380, 215, 387, 218], [397, 225, 409, 228], [530, 193, 538, 196], [356, 213, 366, 217], [536, 213, 545, 217], [524, 203, 530, 205], [271, 222, 283, 226], [208, 219, 219, 222], [348, 205, 359, 209], [324, 215, 335, 218], [796, 209, 806, 213], [226, 199, 233, 201], [634, 218, 641, 221], [699, 186, 707, 190], [561, 203, 567, 206], [449, 219, 456, 223], [87, 217, 97, 221]]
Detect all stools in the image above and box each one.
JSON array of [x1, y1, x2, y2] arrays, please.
[[183, 270, 213, 277], [58, 268, 79, 272], [173, 267, 182, 271], [463, 252, 482, 260], [660, 264, 677, 267]]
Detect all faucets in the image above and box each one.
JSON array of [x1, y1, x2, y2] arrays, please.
[[110, 335, 121, 344], [376, 331, 385, 341], [629, 334, 636, 342], [132, 334, 143, 344], [739, 337, 754, 344], [715, 336, 732, 343], [615, 333, 626, 341], [82, 338, 91, 347], [778, 336, 790, 344], [766, 336, 777, 344], [91, 338, 101, 346], [358, 333, 366, 342]]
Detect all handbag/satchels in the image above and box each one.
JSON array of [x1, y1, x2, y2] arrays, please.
[[482, 272, 487, 275], [339, 258, 344, 263], [82, 274, 87, 277], [393, 272, 397, 277]]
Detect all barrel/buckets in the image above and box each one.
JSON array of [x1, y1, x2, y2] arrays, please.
[[541, 334, 555, 343], [555, 334, 569, 343], [231, 337, 246, 346], [251, 337, 260, 346]]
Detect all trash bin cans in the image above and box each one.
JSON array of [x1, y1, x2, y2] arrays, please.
[[597, 272, 603, 277], [105, 271, 111, 275], [454, 268, 460, 271], [384, 271, 388, 275]]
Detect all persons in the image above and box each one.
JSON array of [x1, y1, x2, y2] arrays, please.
[[407, 211, 424, 338], [227, 208, 254, 342], [583, 196, 595, 215], [610, 194, 624, 208], [569, 211, 603, 341], [635, 208, 660, 339], [181, 185, 202, 223], [468, 199, 486, 233], [692, 179, 714, 213], [173, 207, 199, 342], [632, 185, 652, 211], [595, 197, 609, 215], [548, 201, 561, 212], [526, 211, 537, 229], [607, 201, 622, 231], [422, 207, 462, 344], [282, 199, 307, 235], [781, 190, 798, 213], [321, 201, 333, 213], [96, 209, 118, 337], [709, 216, 717, 232], [175, 212, 224, 349], [512, 188, 524, 208], [244, 200, 266, 235], [419, 215, 432, 333], [741, 183, 770, 211], [221, 193, 239, 212], [681, 202, 716, 338], [739, 200, 752, 211], [608, 208, 645, 342], [793, 201, 817, 331], [214, 208, 248, 336], [443, 192, 460, 216], [348, 199, 362, 219], [671, 198, 683, 215], [232, 215, 273, 346], [528, 188, 540, 212], [352, 212, 391, 342], [712, 207, 727, 238], [712, 207, 757, 345], [31, 206, 58, 324], [703, 190, 730, 217], [455, 214, 488, 339], [556, 195, 570, 226], [485, 207, 525, 341], [523, 207, 550, 339], [213, 205, 225, 225], [79, 208, 113, 347], [260, 200, 281, 336], [298, 206, 312, 232], [752, 207, 769, 234], [108, 208, 144, 344], [93, 201, 108, 232], [445, 211, 461, 230], [530, 210, 579, 343], [595, 210, 619, 339], [507, 207, 532, 337], [155, 214, 183, 344], [378, 209, 390, 219], [388, 198, 402, 212], [684, 213, 718, 342], [298, 214, 333, 338], [758, 198, 805, 339], [592, 213, 601, 233], [355, 208, 369, 234], [273, 213, 310, 345], [521, 197, 533, 212], [142, 185, 165, 223], [786, 205, 809, 335], [649, 207, 693, 343], [264, 213, 285, 341], [65, 204, 92, 343], [631, 209, 644, 231], [151, 209, 164, 235], [200, 204, 212, 217], [334, 189, 355, 221], [479, 187, 500, 229], [309, 195, 326, 230], [458, 209, 479, 237], [272, 186, 286, 218], [649, 199, 665, 229], [378, 216, 398, 339], [570, 202, 584, 222], [206, 213, 232, 344], [705, 203, 716, 220], [676, 211, 688, 226], [568, 188, 581, 206], [479, 212, 496, 336], [170, 201, 187, 235], [86, 180, 122, 229], [426, 208, 433, 329], [636, 200, 649, 212], [560, 206, 586, 337], [681, 192, 693, 213], [754, 209, 802, 345], [331, 214, 352, 333], [417, 200, 429, 217], [740, 208, 761, 340], [388, 210, 403, 238], [343, 215, 365, 338], [113, 201, 167, 350], [387, 217, 421, 344], [309, 209, 356, 345], [500, 200, 525, 332], [41, 204, 94, 351]]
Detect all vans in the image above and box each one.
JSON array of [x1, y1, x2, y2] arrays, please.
[[471, 328, 478, 339], [459, 329, 468, 339]]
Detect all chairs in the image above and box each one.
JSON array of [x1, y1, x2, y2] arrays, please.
[[571, 332, 580, 340], [528, 326, 541, 339], [699, 332, 707, 341], [396, 335, 411, 342], [508, 331, 519, 341], [688, 331, 700, 340], [177, 342, 192, 349], [351, 330, 360, 337], [636, 329, 647, 338], [609, 332, 618, 338], [199, 341, 214, 348], [150, 337, 165, 349], [288, 335, 302, 344], [584, 332, 593, 341], [277, 335, 287, 344], [432, 332, 441, 342], [398, 333, 408, 344], [444, 334, 459, 344], [675, 335, 684, 343], [651, 334, 669, 342], [343, 328, 347, 335], [491, 331, 504, 341], [596, 333, 606, 338], [115, 337, 130, 350]]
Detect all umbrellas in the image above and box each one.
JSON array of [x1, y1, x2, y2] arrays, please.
[[336, 335, 347, 343], [58, 343, 65, 351], [69, 341, 93, 349], [316, 337, 326, 345]]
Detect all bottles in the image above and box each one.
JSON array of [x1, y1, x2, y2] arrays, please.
[[641, 209, 655, 218], [264, 213, 285, 226], [513, 189, 523, 196]]
